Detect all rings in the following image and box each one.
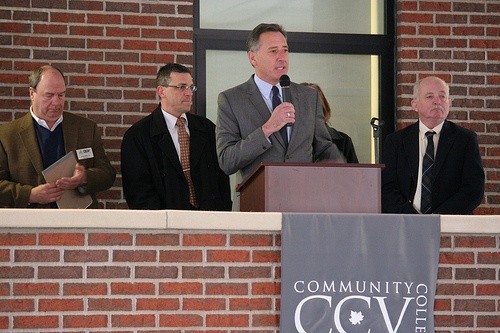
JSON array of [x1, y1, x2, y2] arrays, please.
[[288, 112, 291, 118]]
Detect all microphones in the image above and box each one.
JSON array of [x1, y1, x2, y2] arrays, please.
[[279, 74, 294, 125]]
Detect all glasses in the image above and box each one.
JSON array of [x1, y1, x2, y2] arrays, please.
[[162, 83, 197, 93]]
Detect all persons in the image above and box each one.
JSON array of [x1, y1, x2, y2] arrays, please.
[[0, 64, 116, 209], [300, 83, 358, 164], [121, 63, 233, 211], [216, 24, 344, 178], [383, 76, 485, 215]]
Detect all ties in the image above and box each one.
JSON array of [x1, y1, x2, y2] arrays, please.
[[176, 116, 200, 210], [271, 85, 288, 149], [420, 130, 436, 214]]
